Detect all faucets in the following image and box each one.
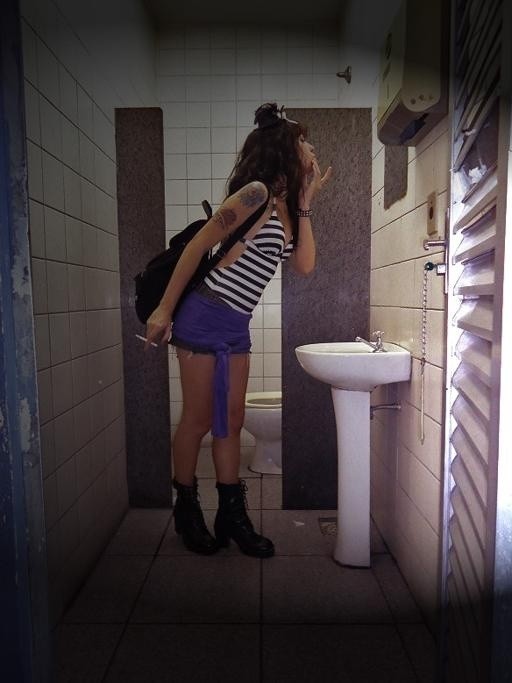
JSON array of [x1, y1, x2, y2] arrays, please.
[[355, 331, 389, 353]]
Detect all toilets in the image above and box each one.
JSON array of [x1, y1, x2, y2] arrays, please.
[[242, 391, 283, 475]]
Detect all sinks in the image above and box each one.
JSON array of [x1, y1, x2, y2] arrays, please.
[[295, 341, 411, 391]]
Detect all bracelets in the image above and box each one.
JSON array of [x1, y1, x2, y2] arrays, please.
[[296, 208, 312, 217]]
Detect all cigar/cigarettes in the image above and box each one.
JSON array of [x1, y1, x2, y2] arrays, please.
[[135, 334, 158, 347]]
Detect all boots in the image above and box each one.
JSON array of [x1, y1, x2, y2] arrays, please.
[[212, 480, 276, 559], [171, 473, 221, 557]]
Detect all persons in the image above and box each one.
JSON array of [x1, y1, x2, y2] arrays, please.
[[144, 118, 333, 557]]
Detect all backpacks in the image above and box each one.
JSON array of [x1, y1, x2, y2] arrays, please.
[[132, 179, 271, 324]]
